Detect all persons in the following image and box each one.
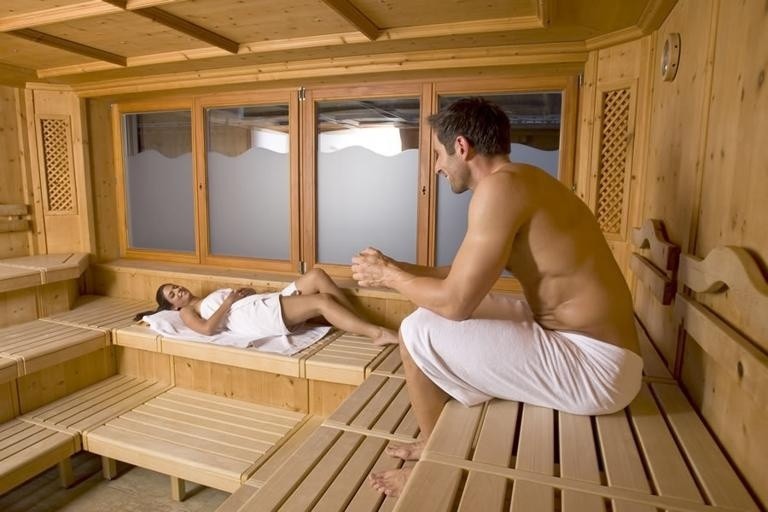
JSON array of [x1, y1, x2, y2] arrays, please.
[[350, 94, 647, 500], [132, 267, 398, 347]]
